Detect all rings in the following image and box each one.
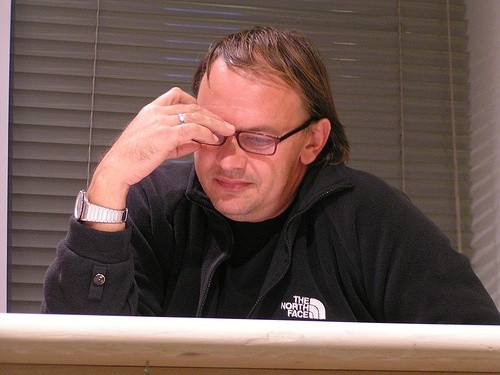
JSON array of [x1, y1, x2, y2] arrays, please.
[[177, 113, 184, 123]]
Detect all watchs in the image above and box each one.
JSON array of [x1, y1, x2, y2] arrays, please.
[[73, 188, 129, 223]]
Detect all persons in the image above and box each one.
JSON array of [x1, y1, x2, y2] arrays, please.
[[39, 24, 500, 326]]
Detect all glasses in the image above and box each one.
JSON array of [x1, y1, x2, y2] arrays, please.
[[192, 117, 318, 155]]
